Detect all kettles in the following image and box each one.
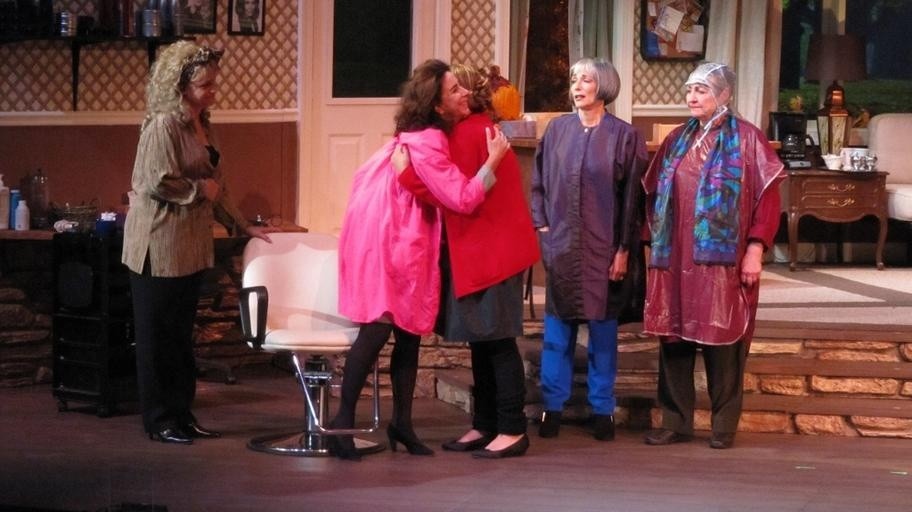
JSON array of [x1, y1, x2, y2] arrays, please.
[[780, 131, 815, 159]]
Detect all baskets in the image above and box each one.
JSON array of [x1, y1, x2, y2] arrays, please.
[[48, 197, 104, 236]]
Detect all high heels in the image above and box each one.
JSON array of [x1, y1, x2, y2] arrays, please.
[[386, 422, 437, 457], [319, 423, 361, 461]]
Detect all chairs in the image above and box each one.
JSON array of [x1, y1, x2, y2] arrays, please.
[[867, 113, 912, 222], [239, 232, 386, 457]]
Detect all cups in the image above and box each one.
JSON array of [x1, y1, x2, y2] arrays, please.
[[821, 147, 876, 170], [95, 220, 116, 239]]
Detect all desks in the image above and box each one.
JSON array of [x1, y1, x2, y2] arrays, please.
[[507, 137, 781, 319]]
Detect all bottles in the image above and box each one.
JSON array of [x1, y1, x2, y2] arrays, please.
[[0, 168, 49, 231]]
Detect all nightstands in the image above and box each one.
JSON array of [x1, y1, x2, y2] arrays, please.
[[762, 170, 889, 271]]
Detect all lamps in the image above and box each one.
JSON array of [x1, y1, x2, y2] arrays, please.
[[816, 80, 848, 155]]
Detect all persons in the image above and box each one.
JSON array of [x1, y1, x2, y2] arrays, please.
[[527, 58, 651, 440], [641, 62, 788, 448], [235, 1, 260, 34], [121, 38, 281, 446], [321, 57, 513, 461], [390, 66, 543, 460]]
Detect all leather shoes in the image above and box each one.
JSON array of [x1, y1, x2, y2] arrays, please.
[[182, 419, 222, 439], [472, 431, 529, 460], [441, 425, 497, 452], [149, 424, 194, 445]]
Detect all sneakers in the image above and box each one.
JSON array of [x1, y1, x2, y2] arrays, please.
[[592, 414, 616, 442], [643, 425, 694, 445], [709, 432, 736, 449], [538, 410, 561, 438]]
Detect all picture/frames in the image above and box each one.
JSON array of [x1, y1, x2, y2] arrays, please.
[[228, 0, 266, 36], [176, 0, 217, 33]]
